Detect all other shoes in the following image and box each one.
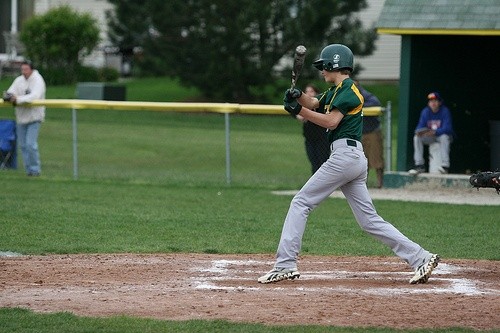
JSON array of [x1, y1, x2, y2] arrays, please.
[[410, 167, 425, 174]]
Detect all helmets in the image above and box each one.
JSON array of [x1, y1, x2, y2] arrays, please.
[[313, 44, 354, 71]]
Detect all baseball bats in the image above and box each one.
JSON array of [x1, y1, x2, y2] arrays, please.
[[290, 45, 307, 91]]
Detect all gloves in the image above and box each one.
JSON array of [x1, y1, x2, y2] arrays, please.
[[285, 90, 301, 99], [284, 101, 302, 115]]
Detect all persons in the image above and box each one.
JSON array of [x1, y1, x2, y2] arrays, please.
[[3, 61, 47, 176], [413, 92, 454, 173], [295, 84, 330, 175], [258, 46, 440, 284], [358, 86, 385, 189]]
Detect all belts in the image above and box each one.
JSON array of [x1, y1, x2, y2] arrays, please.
[[346, 139, 356, 146]]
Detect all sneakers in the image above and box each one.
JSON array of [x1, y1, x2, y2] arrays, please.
[[258, 266, 300, 284], [409, 254, 441, 284]]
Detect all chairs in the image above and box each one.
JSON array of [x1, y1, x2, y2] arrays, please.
[[0, 117, 18, 169]]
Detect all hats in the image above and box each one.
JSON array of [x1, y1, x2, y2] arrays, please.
[[427, 93, 440, 102]]
